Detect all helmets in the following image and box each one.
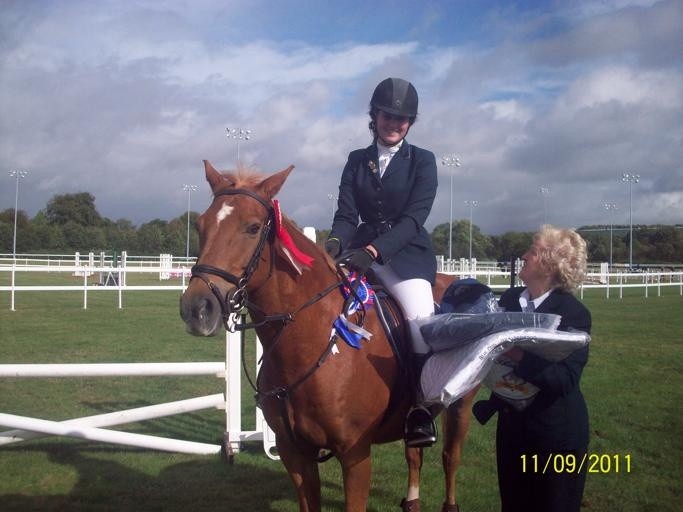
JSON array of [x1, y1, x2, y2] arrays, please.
[[370, 78, 418, 117], [440, 278, 491, 313]]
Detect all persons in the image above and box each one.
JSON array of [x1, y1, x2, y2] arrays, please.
[[323, 76, 437, 446], [471, 222, 591, 512]]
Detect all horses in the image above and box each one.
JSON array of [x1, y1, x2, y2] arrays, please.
[[178, 158, 479, 512]]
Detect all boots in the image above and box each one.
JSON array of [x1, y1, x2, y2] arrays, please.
[[406, 346, 435, 448]]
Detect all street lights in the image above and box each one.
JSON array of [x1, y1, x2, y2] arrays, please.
[[325, 193, 338, 221], [182, 182, 198, 265], [440, 153, 462, 260], [537, 184, 553, 225], [619, 170, 641, 273], [601, 198, 618, 273], [461, 199, 479, 261], [224, 127, 253, 165], [8, 168, 27, 259]]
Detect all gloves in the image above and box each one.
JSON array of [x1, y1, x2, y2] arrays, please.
[[344, 249, 375, 274], [325, 237, 341, 259]]
